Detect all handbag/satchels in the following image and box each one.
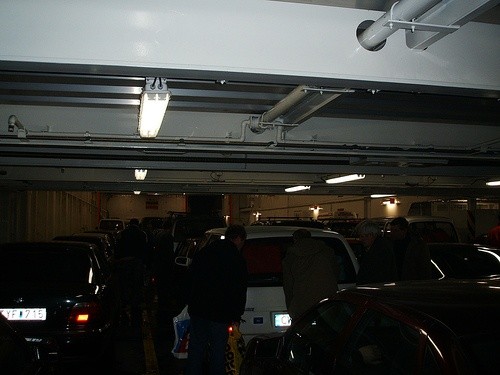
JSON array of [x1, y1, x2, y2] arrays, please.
[[171, 305, 191, 359], [224, 322, 243, 375]]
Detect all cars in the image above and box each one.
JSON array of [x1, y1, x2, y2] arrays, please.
[[54, 230, 115, 268], [177, 224, 363, 358], [238, 278, 500, 375], [425, 242, 500, 280], [0, 238, 135, 375], [353, 214, 462, 245], [96, 217, 125, 236], [143, 217, 228, 247]]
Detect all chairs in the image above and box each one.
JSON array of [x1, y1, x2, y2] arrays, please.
[[432, 228, 448, 241], [359, 326, 401, 358], [243, 244, 285, 273]]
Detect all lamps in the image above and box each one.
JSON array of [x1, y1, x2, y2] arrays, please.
[[321, 173, 366, 184], [284, 185, 311, 193], [137, 76, 172, 140]]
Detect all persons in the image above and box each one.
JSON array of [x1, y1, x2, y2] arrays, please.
[[390, 217, 419, 280], [184, 225, 248, 375], [355, 220, 398, 284], [119, 219, 152, 307], [487, 213, 500, 250], [282, 229, 337, 360]]
